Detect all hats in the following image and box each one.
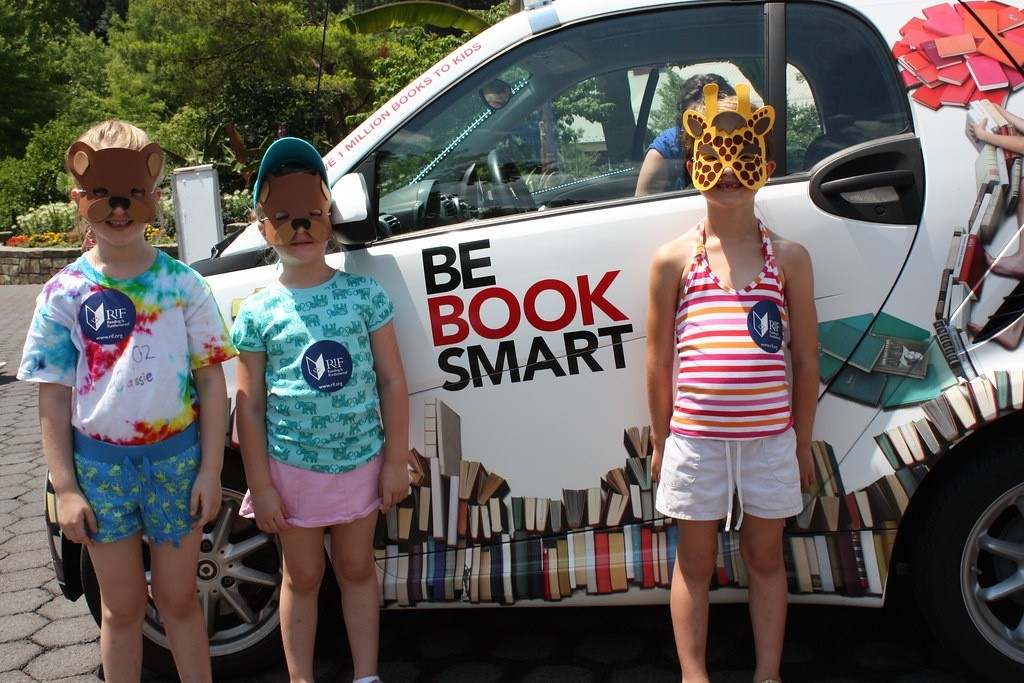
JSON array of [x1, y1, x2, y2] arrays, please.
[[253, 137, 332, 211]]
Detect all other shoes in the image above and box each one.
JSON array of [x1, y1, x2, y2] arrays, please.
[[353, 675, 380, 683]]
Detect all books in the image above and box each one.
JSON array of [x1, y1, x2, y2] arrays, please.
[[373, 397, 749, 607], [782, 100, 1022, 599], [892, 0, 1024, 111]]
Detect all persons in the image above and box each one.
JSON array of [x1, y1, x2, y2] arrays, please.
[[17, 121, 241, 683], [230, 137, 411, 683], [968, 103, 1024, 277], [634, 75, 735, 198], [647, 101, 820, 683]]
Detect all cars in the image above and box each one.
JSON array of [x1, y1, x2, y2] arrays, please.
[[43, 0, 1024, 683]]
